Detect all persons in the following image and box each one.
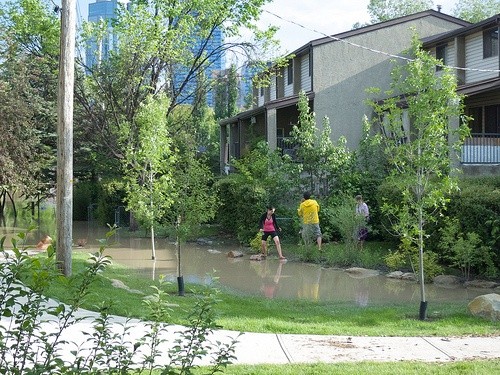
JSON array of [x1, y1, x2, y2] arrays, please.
[[258, 205, 286, 259], [260, 260, 285, 299], [353, 195, 371, 251], [297, 264, 321, 301], [297, 192, 324, 252]]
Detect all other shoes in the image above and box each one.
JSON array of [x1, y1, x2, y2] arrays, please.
[[279, 256, 287, 260]]
